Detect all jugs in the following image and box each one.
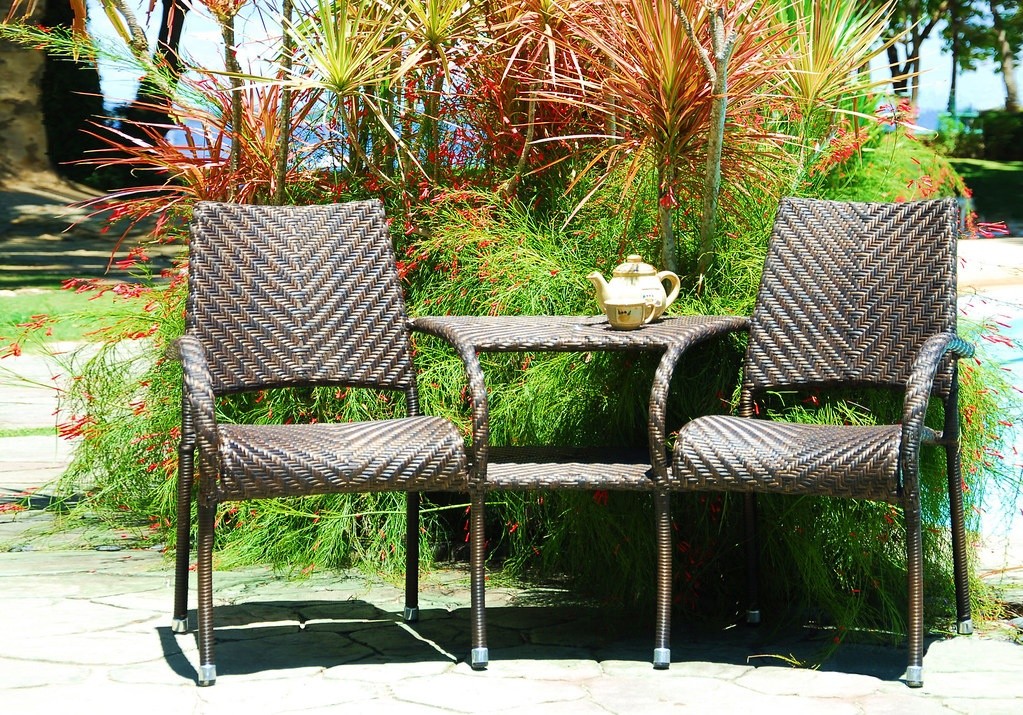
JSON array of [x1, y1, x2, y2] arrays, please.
[[586, 254, 682, 322]]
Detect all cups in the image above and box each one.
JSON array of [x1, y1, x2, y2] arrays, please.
[[603, 298, 656, 330]]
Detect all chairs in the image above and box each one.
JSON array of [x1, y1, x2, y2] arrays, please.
[[648, 193, 974, 689], [172, 200, 490, 686]]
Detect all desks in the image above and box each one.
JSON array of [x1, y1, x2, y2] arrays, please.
[[421, 316, 757, 493]]
